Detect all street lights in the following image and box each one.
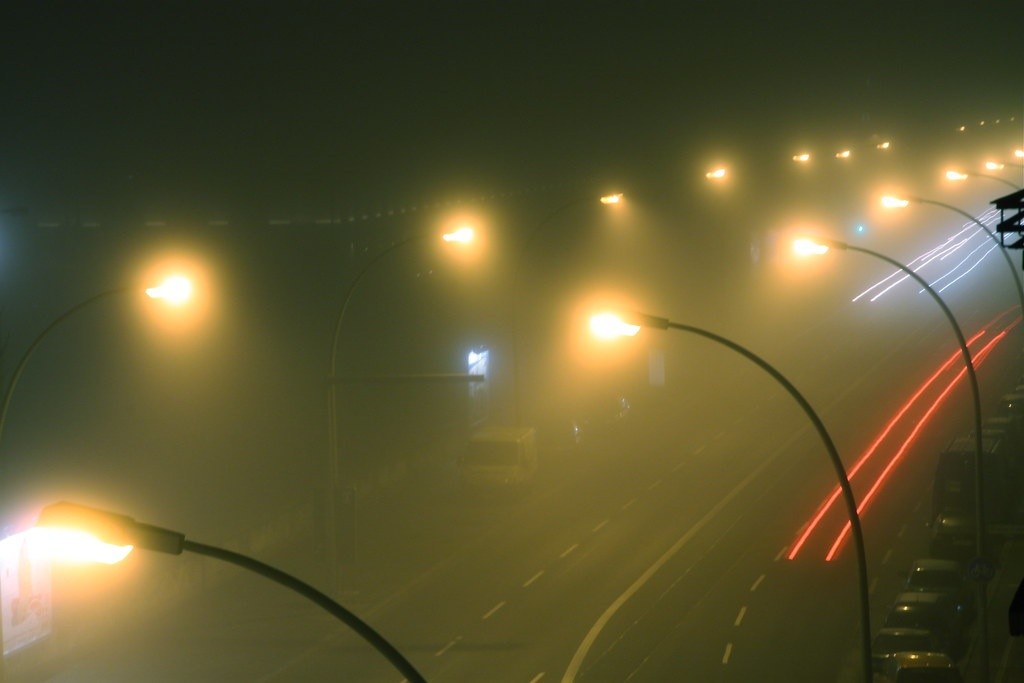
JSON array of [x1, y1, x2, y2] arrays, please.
[[792, 232, 985, 573], [945, 169, 1023, 199], [878, 191, 1024, 317], [329, 225, 477, 551], [38, 502, 426, 683], [986, 161, 1023, 172], [0, 272, 198, 425], [589, 291, 874, 683], [513, 190, 630, 425]]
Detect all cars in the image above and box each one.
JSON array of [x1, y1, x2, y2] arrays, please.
[[876, 651, 963, 683], [870, 624, 933, 678], [885, 590, 946, 631], [906, 559, 970, 606], [929, 380, 1024, 557]]
